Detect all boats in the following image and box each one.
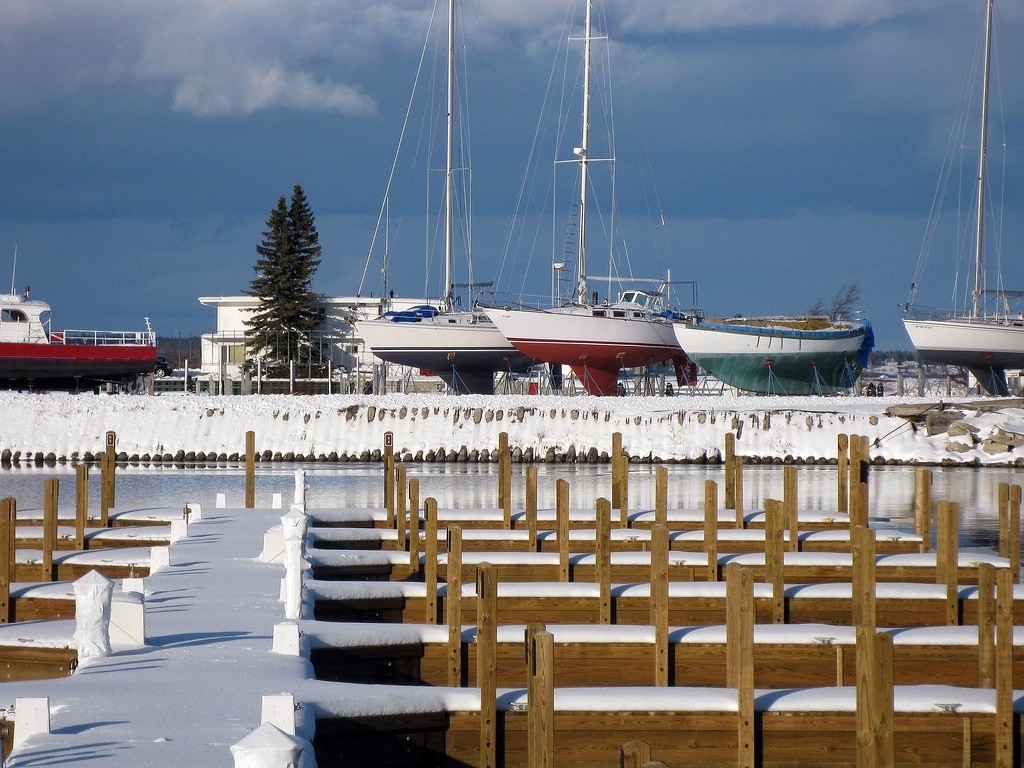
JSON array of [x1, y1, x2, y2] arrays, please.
[[672, 279, 875, 396], [0, 244, 157, 392]]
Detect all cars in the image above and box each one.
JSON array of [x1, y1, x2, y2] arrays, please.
[[154, 356, 173, 378], [157, 357, 168, 362]]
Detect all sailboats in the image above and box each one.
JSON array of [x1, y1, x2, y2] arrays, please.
[[898, 1, 1024, 397], [349, 0, 562, 395], [476, 0, 705, 397]]
